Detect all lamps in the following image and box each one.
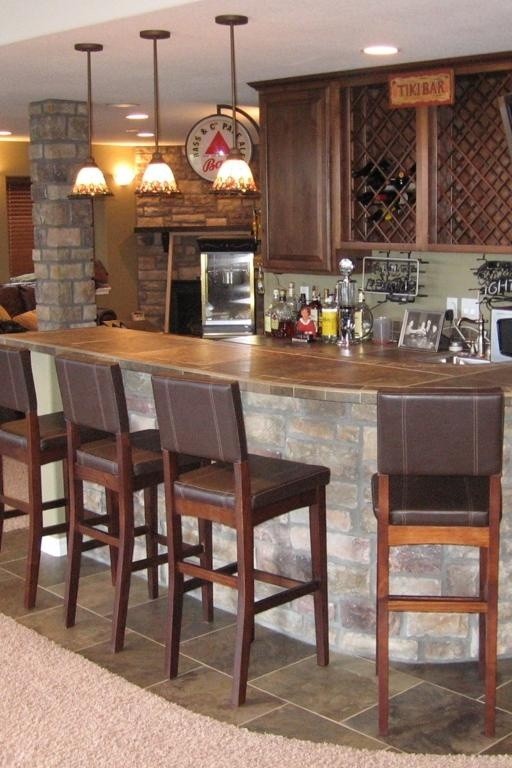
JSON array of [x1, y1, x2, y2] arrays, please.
[[211, 13, 260, 197], [67, 43, 114, 199], [116, 163, 136, 191], [138, 29, 181, 198]]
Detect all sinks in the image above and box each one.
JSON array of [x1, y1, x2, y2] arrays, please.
[[417, 353, 492, 367]]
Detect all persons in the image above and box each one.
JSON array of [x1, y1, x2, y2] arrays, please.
[[295, 306, 317, 339], [405, 318, 438, 344]]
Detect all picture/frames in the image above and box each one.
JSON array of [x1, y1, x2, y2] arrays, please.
[[397, 306, 445, 355]]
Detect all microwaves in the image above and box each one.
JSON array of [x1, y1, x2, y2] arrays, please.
[[489, 306, 512, 364]]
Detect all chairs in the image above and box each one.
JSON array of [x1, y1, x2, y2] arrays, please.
[[0, 345, 115, 610], [147, 370, 333, 709], [370, 385, 504, 739], [54, 351, 215, 657]]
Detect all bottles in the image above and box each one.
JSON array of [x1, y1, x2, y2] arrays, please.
[[287, 282, 298, 331], [359, 181, 372, 202], [395, 171, 408, 187], [296, 291, 307, 321], [409, 199, 416, 200], [321, 294, 338, 343], [309, 286, 321, 335], [264, 289, 280, 337], [353, 288, 371, 342], [322, 290, 330, 304], [271, 290, 292, 337], [374, 193, 399, 203], [364, 205, 401, 223], [333, 284, 342, 304]]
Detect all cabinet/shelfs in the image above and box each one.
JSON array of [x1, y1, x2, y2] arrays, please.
[[245, 50, 512, 276]]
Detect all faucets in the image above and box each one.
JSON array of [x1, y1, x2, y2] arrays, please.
[[454, 312, 489, 357]]
